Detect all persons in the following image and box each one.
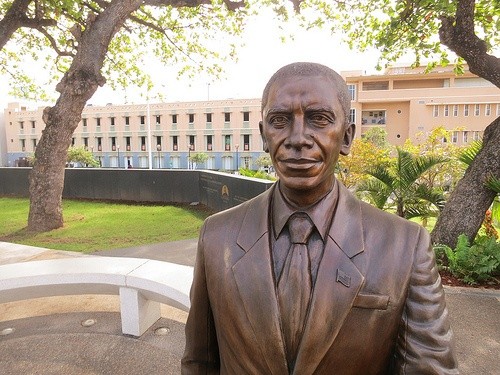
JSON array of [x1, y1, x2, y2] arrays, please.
[[181, 61, 461, 375]]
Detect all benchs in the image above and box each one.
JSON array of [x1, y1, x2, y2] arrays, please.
[[0, 257, 194, 337]]
